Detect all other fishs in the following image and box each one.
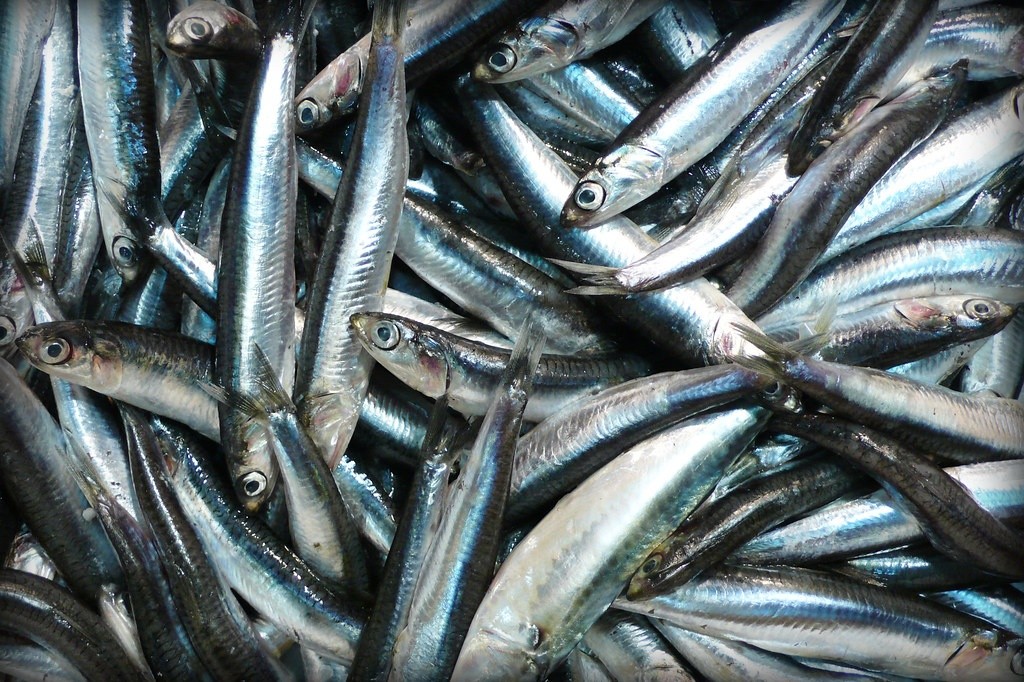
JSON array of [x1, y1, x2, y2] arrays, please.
[[0, 0, 1024, 682]]
